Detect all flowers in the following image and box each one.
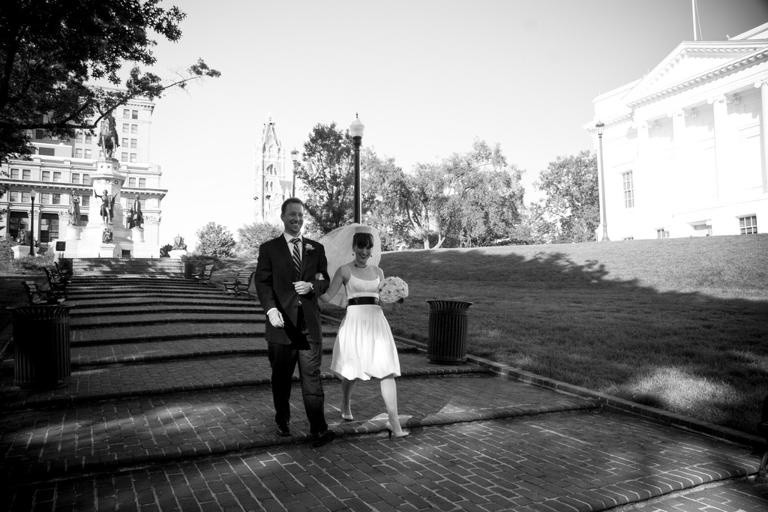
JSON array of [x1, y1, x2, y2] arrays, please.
[[377, 276, 408, 304]]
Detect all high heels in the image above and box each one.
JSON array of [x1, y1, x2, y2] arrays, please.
[[386, 420, 410, 439], [341, 412, 354, 421]]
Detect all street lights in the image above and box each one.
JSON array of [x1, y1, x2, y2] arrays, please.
[[595, 120, 610, 241], [348, 111, 366, 223], [29, 189, 36, 257], [290, 150, 300, 197]]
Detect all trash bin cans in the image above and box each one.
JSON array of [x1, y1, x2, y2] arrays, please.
[[426, 300, 473, 365], [5, 305, 76, 389], [184, 261, 194, 278], [58, 258, 74, 275]]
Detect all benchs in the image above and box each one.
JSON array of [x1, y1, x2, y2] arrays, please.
[[221, 271, 253, 298], [192, 264, 215, 284], [21, 262, 70, 305]]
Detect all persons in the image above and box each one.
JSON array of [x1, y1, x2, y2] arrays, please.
[[68, 189, 82, 226], [97, 108, 121, 148], [93, 188, 118, 224], [315, 231, 409, 439], [101, 228, 113, 243], [253, 197, 336, 449], [129, 193, 145, 228]]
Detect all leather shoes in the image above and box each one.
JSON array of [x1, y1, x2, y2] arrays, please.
[[311, 430, 336, 447], [277, 422, 289, 436]]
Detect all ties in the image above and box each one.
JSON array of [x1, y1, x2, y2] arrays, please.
[[290, 238, 302, 279]]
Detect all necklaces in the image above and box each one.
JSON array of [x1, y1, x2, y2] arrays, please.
[[352, 259, 368, 269]]
